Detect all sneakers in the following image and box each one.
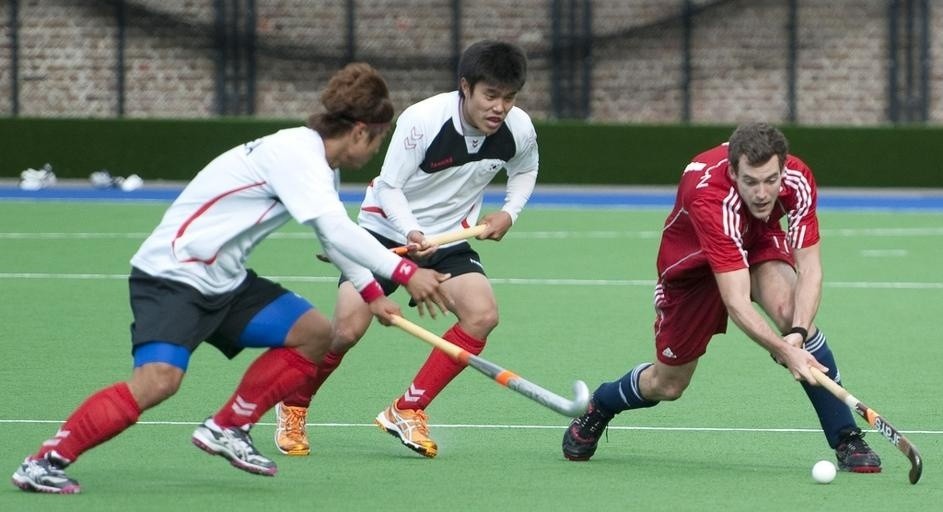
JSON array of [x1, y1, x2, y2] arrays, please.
[[562, 382, 615, 462], [374, 398, 439, 459], [191, 400, 311, 477], [11, 449, 81, 494], [835, 427, 882, 473]]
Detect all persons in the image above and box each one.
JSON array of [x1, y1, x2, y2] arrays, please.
[[559, 120, 885, 472], [7, 61, 456, 496], [269, 42, 545, 458]]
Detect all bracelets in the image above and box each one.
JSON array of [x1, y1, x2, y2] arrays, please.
[[785, 325, 809, 339], [358, 280, 385, 302], [389, 256, 417, 287]]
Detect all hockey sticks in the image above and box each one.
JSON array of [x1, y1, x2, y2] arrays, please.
[[383, 312, 592, 418], [810, 367, 922, 485], [316, 226, 490, 265]]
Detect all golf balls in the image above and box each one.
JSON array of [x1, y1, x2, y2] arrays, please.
[[811, 462, 838, 483]]
